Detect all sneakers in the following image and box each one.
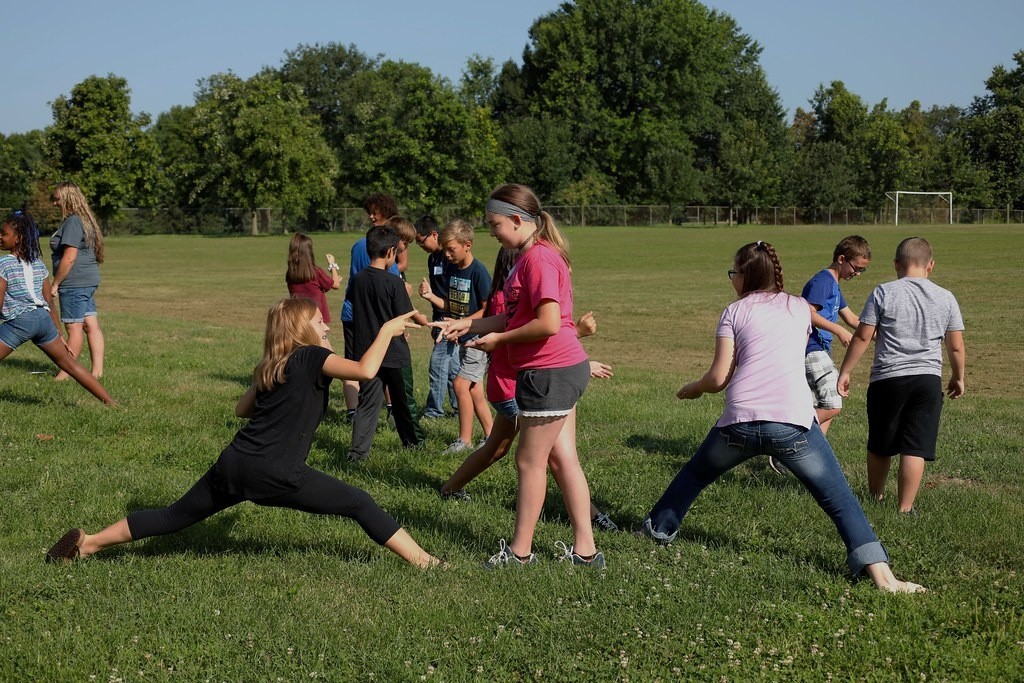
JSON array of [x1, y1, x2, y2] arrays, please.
[[549, 540, 605, 569], [486, 539, 537, 567]]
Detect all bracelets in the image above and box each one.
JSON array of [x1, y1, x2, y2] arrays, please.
[[328, 263, 339, 272]]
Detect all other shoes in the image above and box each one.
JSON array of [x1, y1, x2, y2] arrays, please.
[[439, 440, 474, 457], [444, 490, 469, 498], [477, 439, 490, 447], [880, 582, 925, 594], [646, 518, 678, 545], [769, 455, 787, 478], [593, 513, 619, 533]]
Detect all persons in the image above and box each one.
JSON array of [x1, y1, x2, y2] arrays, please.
[[426, 184, 607, 569], [414, 215, 462, 419], [50, 183, 105, 380], [44, 296, 455, 571], [637, 239, 927, 596], [768, 235, 877, 475], [346, 224, 428, 462], [359, 192, 409, 282], [340, 216, 418, 424], [439, 245, 619, 533], [419, 219, 493, 454], [837, 236, 965, 513], [0, 210, 117, 407], [285, 233, 340, 324]]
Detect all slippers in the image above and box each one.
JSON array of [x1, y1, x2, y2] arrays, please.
[[46, 528, 84, 566]]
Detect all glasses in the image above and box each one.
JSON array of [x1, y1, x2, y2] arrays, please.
[[415, 236, 426, 246], [728, 269, 739, 280], [843, 257, 865, 274]]
